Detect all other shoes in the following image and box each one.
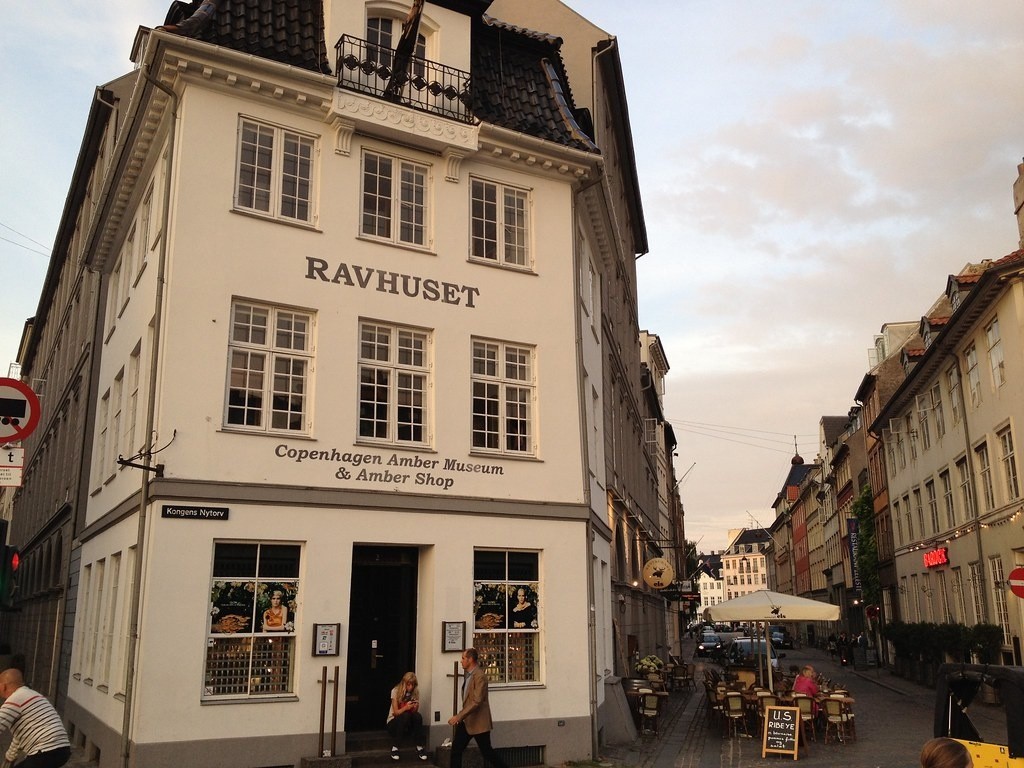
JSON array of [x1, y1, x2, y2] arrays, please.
[[391, 747, 400, 760], [414, 747, 429, 760]]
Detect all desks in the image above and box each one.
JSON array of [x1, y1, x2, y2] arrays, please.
[[624, 679, 668, 697]]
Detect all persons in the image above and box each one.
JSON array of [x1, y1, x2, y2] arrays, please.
[[261, 589, 287, 633], [0, 669, 72, 768], [828, 630, 867, 647], [513, 589, 536, 628], [386, 672, 427, 760], [796, 630, 801, 649], [447, 648, 497, 768], [789, 665, 820, 741], [689, 625, 693, 635], [919, 737, 974, 768]]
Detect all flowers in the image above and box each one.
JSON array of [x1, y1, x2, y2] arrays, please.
[[633, 654, 664, 673]]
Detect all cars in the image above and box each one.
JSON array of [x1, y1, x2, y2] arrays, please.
[[737, 625, 757, 637], [723, 636, 787, 673], [698, 634, 725, 658], [771, 632, 794, 650], [700, 624, 733, 638]]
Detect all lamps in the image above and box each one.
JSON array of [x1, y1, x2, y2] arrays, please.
[[992, 579, 1010, 592], [897, 584, 909, 594], [921, 585, 934, 598]]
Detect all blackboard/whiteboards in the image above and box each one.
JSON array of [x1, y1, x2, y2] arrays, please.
[[762, 706, 806, 754]]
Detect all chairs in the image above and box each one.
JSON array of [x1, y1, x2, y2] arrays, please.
[[701, 667, 856, 746], [638, 693, 660, 739], [669, 664, 697, 692]]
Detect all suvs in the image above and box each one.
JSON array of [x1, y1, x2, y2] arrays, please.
[[763, 625, 787, 639]]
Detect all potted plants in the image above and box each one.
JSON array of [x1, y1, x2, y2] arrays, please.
[[884, 622, 1005, 704]]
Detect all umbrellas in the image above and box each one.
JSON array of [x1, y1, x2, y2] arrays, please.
[[703, 588, 840, 692]]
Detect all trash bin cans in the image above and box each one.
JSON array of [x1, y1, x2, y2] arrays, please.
[[621, 678, 649, 705]]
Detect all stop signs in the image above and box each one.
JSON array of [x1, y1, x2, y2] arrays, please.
[[1007, 568, 1024, 598]]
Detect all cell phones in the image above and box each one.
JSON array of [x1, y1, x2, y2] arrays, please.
[[411, 701, 418, 704]]
